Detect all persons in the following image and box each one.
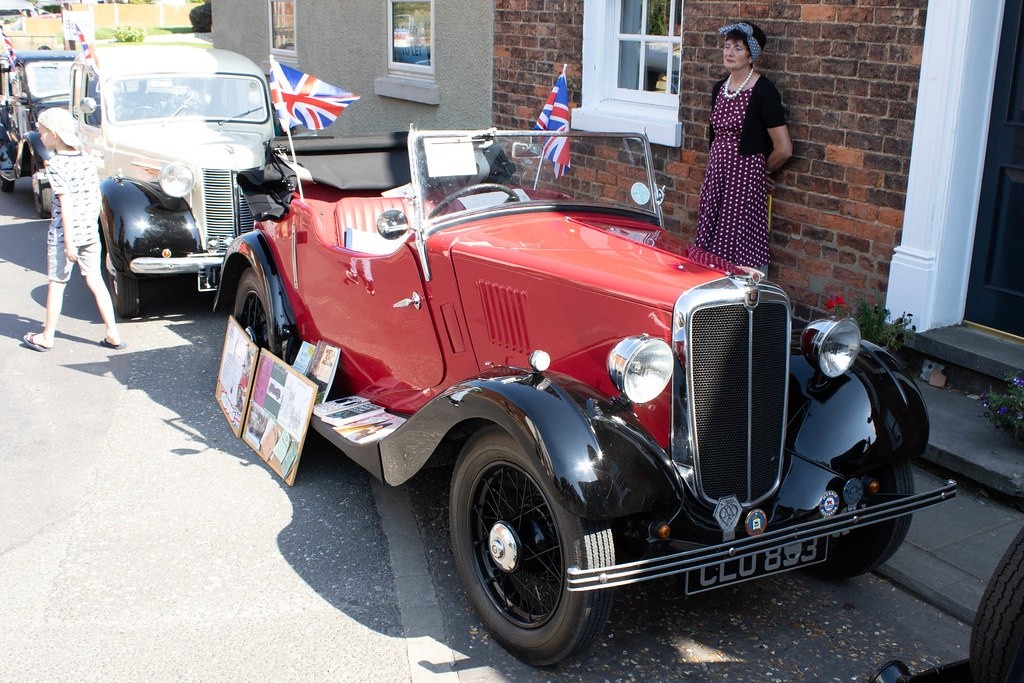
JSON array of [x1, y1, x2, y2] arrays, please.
[[4, 19, 11, 25], [692, 19, 794, 283], [28, 45, 70, 96], [23, 106, 127, 352]]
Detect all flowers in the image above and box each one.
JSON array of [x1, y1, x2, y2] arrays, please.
[[977, 370, 1024, 444], [826, 295, 917, 350]]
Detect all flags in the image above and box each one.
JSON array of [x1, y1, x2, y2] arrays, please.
[[75, 23, 111, 85], [0, 25, 24, 71], [533, 64, 572, 180], [270, 57, 363, 134]]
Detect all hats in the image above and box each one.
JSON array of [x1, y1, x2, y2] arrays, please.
[[37, 107, 84, 146]]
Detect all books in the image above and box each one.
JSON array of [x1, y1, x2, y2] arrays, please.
[[213, 316, 410, 479]]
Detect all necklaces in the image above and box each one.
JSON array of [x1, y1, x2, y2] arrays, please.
[[724, 67, 754, 100]]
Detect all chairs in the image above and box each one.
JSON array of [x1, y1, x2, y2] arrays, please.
[[334, 195, 467, 263]]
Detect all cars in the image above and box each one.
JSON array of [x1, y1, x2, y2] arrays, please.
[[0, 44, 84, 219], [210, 125, 960, 671], [66, 39, 300, 318]]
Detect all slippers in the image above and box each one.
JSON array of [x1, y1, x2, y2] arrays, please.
[[23, 331, 49, 352], [100, 337, 127, 350]]
